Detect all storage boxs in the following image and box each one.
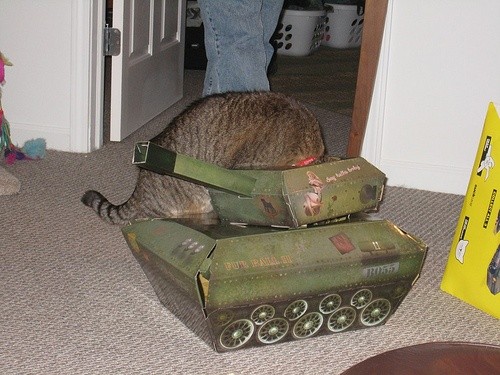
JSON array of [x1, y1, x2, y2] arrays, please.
[[122, 219, 430, 352], [270, 8, 327, 56], [320, 2, 364, 50], [439, 101, 500, 322]]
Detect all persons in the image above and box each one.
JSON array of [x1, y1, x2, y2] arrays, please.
[[197, 1, 283, 97]]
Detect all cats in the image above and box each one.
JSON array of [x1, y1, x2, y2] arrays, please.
[[79, 88, 351, 229]]
